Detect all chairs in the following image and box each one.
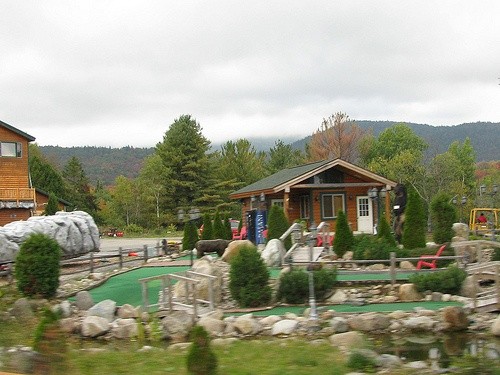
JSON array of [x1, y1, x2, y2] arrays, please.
[[416, 245, 446, 269], [233, 226, 244, 240], [316, 234, 334, 247]]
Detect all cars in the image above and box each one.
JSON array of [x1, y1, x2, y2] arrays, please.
[[199, 219, 242, 239], [101, 227, 123, 237]]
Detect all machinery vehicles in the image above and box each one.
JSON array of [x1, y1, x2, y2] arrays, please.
[[470, 208, 500, 235]]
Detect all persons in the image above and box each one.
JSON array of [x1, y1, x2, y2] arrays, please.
[[392, 208, 405, 245], [476, 213, 487, 223]]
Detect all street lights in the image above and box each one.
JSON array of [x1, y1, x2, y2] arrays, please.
[[479, 183, 486, 208], [177, 207, 201, 266], [250, 191, 266, 244], [367, 185, 386, 227], [448, 194, 458, 206], [491, 182, 497, 208], [459, 195, 467, 223]]
